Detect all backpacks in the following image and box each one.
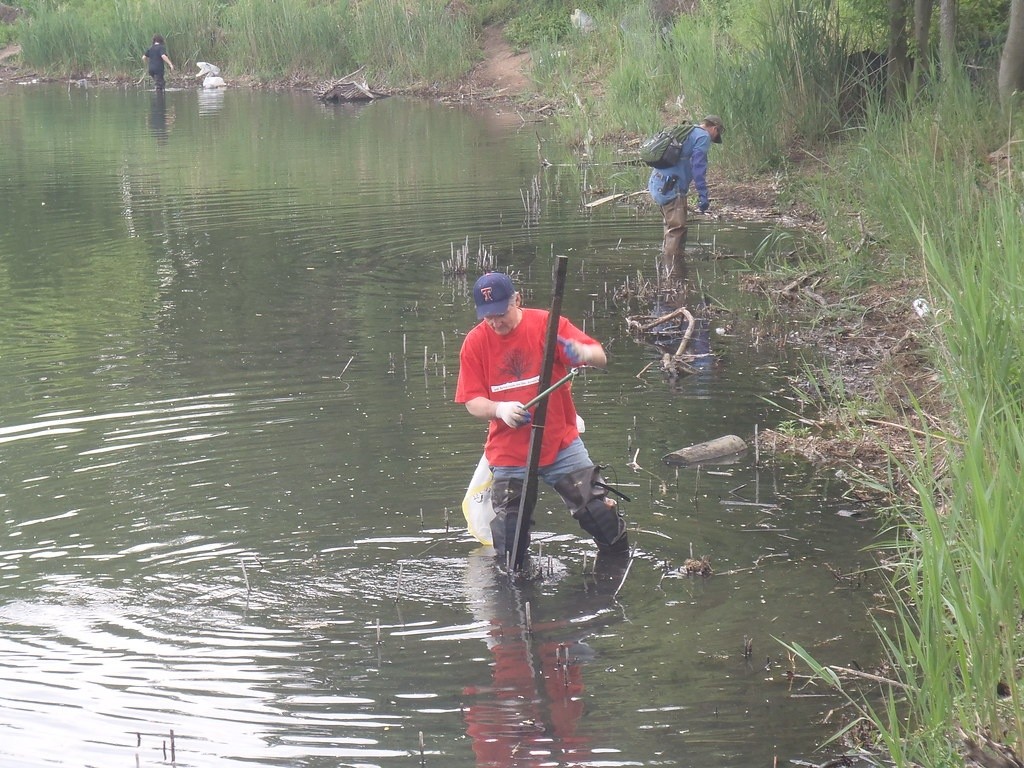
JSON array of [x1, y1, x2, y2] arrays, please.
[[639, 120, 706, 169]]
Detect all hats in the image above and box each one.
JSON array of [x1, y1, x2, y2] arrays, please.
[[474, 273, 514, 320], [704, 115, 725, 144]]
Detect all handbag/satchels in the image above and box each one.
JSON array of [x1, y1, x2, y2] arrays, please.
[[462, 450, 493, 545]]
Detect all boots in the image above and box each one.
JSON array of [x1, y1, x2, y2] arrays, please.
[[660, 196, 688, 282], [489, 477, 539, 555], [553, 465, 627, 554]]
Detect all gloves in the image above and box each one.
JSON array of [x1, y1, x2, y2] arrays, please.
[[699, 196, 709, 212], [557, 334, 592, 364], [496, 402, 532, 428]]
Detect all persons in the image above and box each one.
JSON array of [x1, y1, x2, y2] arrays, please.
[[141, 32, 176, 92], [451, 271, 631, 565], [644, 114, 728, 261]]
